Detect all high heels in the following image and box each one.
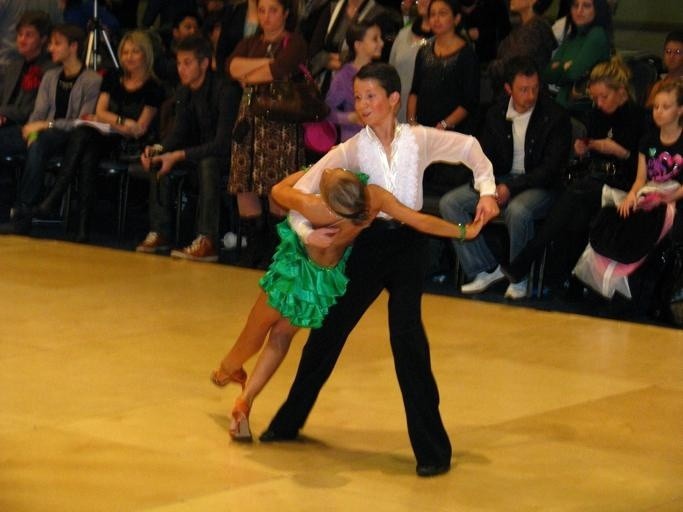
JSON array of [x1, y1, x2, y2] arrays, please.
[[212, 362, 246, 391], [229, 408, 252, 441]]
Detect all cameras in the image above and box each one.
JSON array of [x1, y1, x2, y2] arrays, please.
[[149, 149, 164, 171]]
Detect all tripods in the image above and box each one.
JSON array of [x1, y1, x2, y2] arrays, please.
[[82, 19, 122, 74]]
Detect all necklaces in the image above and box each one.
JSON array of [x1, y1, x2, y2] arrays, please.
[[312, 192, 341, 220]]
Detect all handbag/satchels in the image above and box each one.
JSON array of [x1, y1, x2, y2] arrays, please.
[[249, 58, 331, 124], [568, 150, 618, 190], [590, 200, 666, 264], [303, 119, 337, 153]]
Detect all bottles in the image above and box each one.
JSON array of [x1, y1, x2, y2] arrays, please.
[[147, 144, 164, 172]]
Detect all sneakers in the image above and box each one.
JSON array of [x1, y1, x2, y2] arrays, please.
[[170, 235, 218, 263], [461, 265, 506, 294], [503, 279, 528, 300], [136, 232, 167, 253]]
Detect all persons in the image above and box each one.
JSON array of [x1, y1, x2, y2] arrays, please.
[[458, 0, 498, 58], [572, 77, 682, 324], [550, 1, 572, 47], [167, 14, 205, 53], [656, 33, 682, 80], [545, 0, 619, 108], [506, 54, 638, 311], [322, 21, 386, 147], [214, 0, 265, 71], [32, 30, 162, 245], [405, 1, 477, 131], [381, 2, 415, 41], [480, 0, 559, 106], [0, 9, 59, 231], [206, 164, 484, 440], [258, 62, 500, 478], [438, 58, 571, 302], [134, 31, 243, 262], [389, 1, 440, 127], [220, 0, 311, 273], [0, 24, 101, 240], [301, 1, 401, 96]]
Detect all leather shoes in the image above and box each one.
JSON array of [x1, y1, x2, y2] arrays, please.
[[416, 461, 450, 478], [259, 429, 300, 441]]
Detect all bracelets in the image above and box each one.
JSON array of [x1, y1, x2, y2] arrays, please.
[[46, 119, 54, 130], [439, 119, 447, 129], [458, 221, 466, 242], [407, 116, 418, 122], [116, 115, 127, 128]]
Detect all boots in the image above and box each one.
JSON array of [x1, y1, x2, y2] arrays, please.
[[75, 192, 94, 241], [33, 182, 66, 225]]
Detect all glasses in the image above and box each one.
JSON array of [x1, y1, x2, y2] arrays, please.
[[664, 49, 682, 55]]
